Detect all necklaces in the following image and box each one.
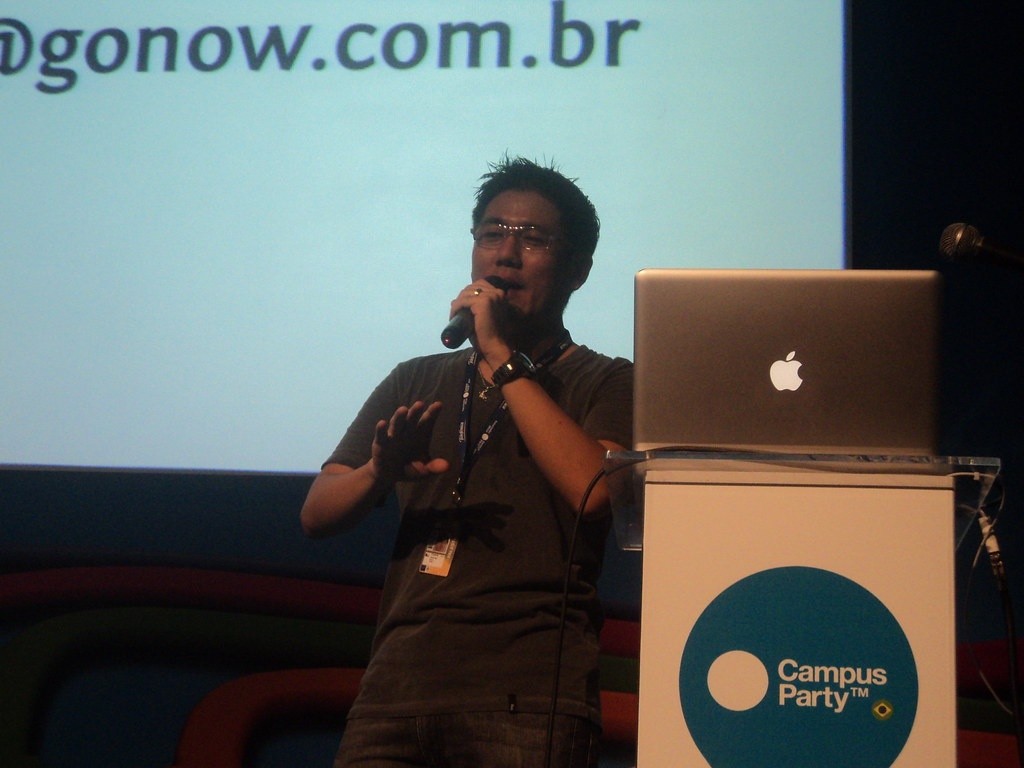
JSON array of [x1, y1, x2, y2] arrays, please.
[[476, 365, 501, 401]]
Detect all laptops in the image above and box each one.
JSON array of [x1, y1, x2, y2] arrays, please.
[[630, 270, 944, 507]]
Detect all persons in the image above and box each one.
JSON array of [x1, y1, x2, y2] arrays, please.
[[297, 162, 635, 768]]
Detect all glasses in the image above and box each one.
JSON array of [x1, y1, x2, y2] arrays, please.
[[470, 222, 559, 255]]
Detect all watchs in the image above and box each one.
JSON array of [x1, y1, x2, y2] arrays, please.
[[490, 350, 538, 388]]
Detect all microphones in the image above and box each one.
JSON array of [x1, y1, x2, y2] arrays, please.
[[441, 276, 508, 349]]
